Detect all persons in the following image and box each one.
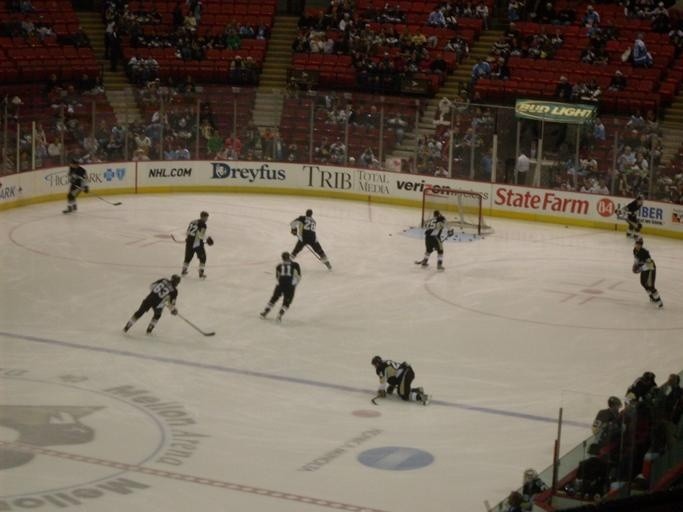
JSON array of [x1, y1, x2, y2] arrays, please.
[[62, 158, 91, 213], [432, 210, 442, 220], [631, 237, 664, 308], [122, 274, 180, 335], [289, 207, 332, 270], [620, 194, 646, 239], [420, 216, 454, 270], [505, 369, 682, 511], [258, 251, 302, 322], [371, 355, 428, 406], [180, 210, 215, 277]]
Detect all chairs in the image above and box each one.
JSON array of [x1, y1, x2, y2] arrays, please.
[[531, 401, 683, 512], [661, 144, 683, 204], [473, 1, 683, 115], [290, 0, 486, 94], [0, 85, 115, 167], [105, 2, 276, 84], [134, 84, 255, 161], [0, 0, 99, 84], [279, 87, 426, 174]]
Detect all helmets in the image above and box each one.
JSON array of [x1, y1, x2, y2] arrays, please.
[[306, 209, 312, 216], [282, 252, 289, 261], [371, 356, 382, 367], [171, 274, 180, 286], [69, 160, 80, 168], [200, 211, 209, 220], [433, 210, 440, 216], [608, 396, 622, 409]]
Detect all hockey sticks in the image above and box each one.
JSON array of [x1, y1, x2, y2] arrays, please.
[[176, 311, 215, 337], [89, 190, 122, 206], [414, 233, 448, 264], [371, 391, 388, 406], [171, 234, 207, 244]]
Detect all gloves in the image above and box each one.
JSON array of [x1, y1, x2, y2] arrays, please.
[[171, 309, 177, 315], [377, 388, 386, 398], [447, 229, 454, 236], [206, 237, 214, 246]]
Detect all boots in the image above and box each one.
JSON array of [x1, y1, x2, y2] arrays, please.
[[63, 204, 77, 213], [421, 258, 428, 266], [146, 329, 151, 334], [198, 269, 206, 277], [627, 227, 640, 239], [412, 387, 430, 405], [123, 326, 129, 332], [181, 267, 188, 275], [260, 307, 270, 317], [324, 260, 332, 269], [278, 309, 284, 320], [649, 293, 663, 307], [437, 260, 445, 269]]
